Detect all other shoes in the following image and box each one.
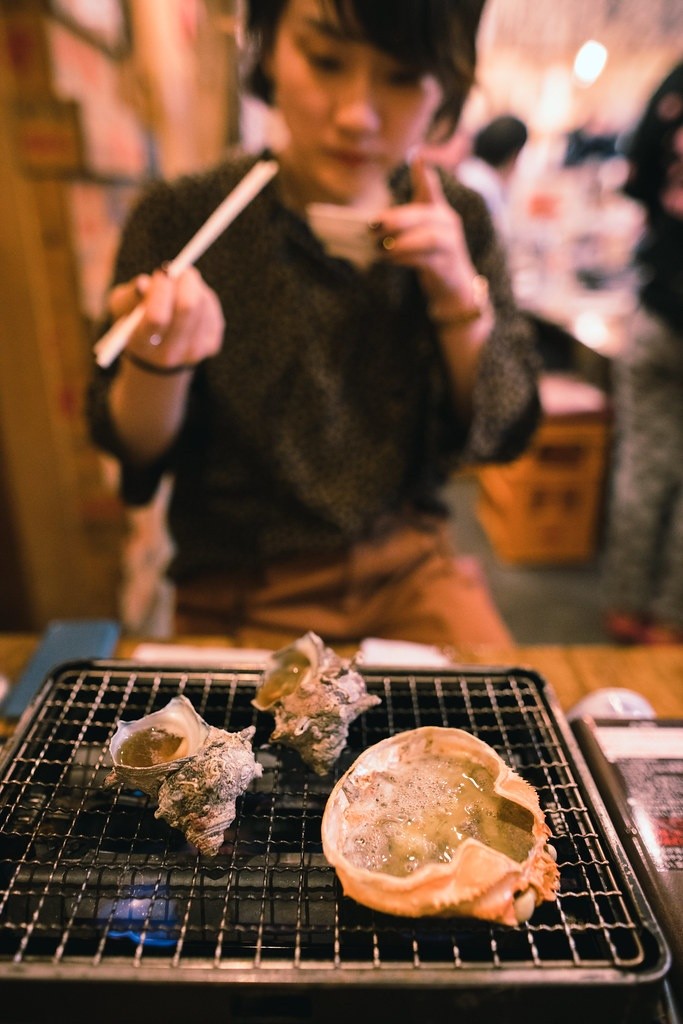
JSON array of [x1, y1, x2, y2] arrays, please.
[[600, 598, 676, 647]]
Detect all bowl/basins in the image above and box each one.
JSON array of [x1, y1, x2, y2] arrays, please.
[[304, 202, 397, 265]]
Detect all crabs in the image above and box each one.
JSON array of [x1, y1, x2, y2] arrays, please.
[[321, 726, 561, 927]]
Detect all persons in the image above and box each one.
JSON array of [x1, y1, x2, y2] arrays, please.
[[82, 2, 543, 642], [451, 58, 683, 643]]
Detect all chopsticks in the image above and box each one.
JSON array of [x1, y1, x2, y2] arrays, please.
[[92, 159, 278, 369]]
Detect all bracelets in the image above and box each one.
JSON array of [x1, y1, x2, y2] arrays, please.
[[424, 276, 489, 327], [121, 342, 195, 378]]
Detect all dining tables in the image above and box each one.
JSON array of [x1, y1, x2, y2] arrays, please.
[[0, 633, 683, 738]]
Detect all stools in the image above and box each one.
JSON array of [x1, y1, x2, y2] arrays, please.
[[470, 368, 608, 564]]
